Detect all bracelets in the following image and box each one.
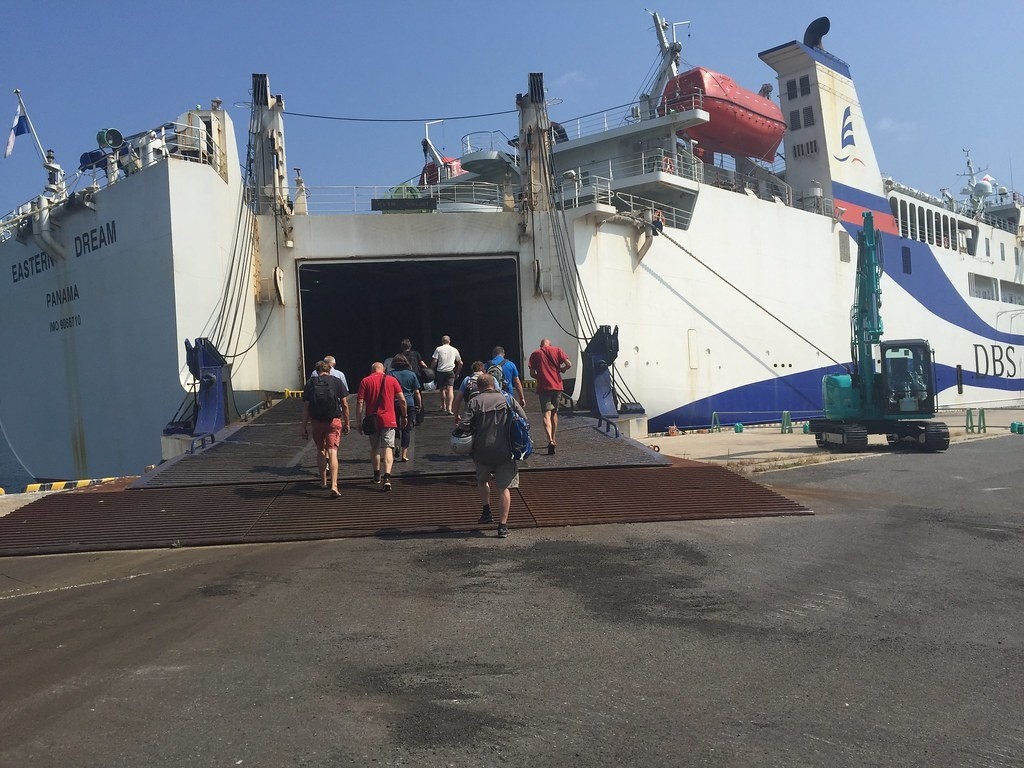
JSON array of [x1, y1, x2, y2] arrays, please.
[[401, 415, 408, 418]]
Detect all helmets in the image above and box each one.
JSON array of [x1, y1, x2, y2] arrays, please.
[[450, 429, 475, 454]]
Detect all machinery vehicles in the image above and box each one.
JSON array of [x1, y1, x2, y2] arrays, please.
[[807, 210, 952, 455]]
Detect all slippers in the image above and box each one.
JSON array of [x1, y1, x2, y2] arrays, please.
[[331, 493, 341, 499], [319, 481, 327, 488]]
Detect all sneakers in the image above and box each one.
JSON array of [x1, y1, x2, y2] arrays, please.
[[498, 527, 512, 537], [478, 512, 494, 523], [382, 479, 392, 491], [373, 474, 380, 483]]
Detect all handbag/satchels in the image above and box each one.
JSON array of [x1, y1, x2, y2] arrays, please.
[[501, 390, 534, 460], [422, 368, 435, 384], [362, 413, 377, 435]]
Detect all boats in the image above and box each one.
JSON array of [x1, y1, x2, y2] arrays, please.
[[656, 66, 788, 165], [0, 8, 1024, 500]]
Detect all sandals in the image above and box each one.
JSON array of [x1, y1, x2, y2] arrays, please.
[[547, 440, 557, 448]]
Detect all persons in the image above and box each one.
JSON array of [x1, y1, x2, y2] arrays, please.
[[528, 338, 571, 455], [383, 338, 427, 461], [356, 362, 408, 491], [311, 356, 349, 470], [301, 361, 350, 497], [430, 335, 463, 415], [454, 346, 527, 538]]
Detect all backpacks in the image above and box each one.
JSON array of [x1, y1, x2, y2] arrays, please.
[[486, 359, 509, 390], [311, 375, 337, 423], [463, 375, 483, 403]]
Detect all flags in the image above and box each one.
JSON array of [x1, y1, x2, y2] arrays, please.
[[5, 102, 32, 158]]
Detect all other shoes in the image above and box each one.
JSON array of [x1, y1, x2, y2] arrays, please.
[[402, 457, 409, 462], [395, 446, 400, 458], [440, 406, 446, 411], [325, 465, 329, 474], [447, 410, 455, 415]]
